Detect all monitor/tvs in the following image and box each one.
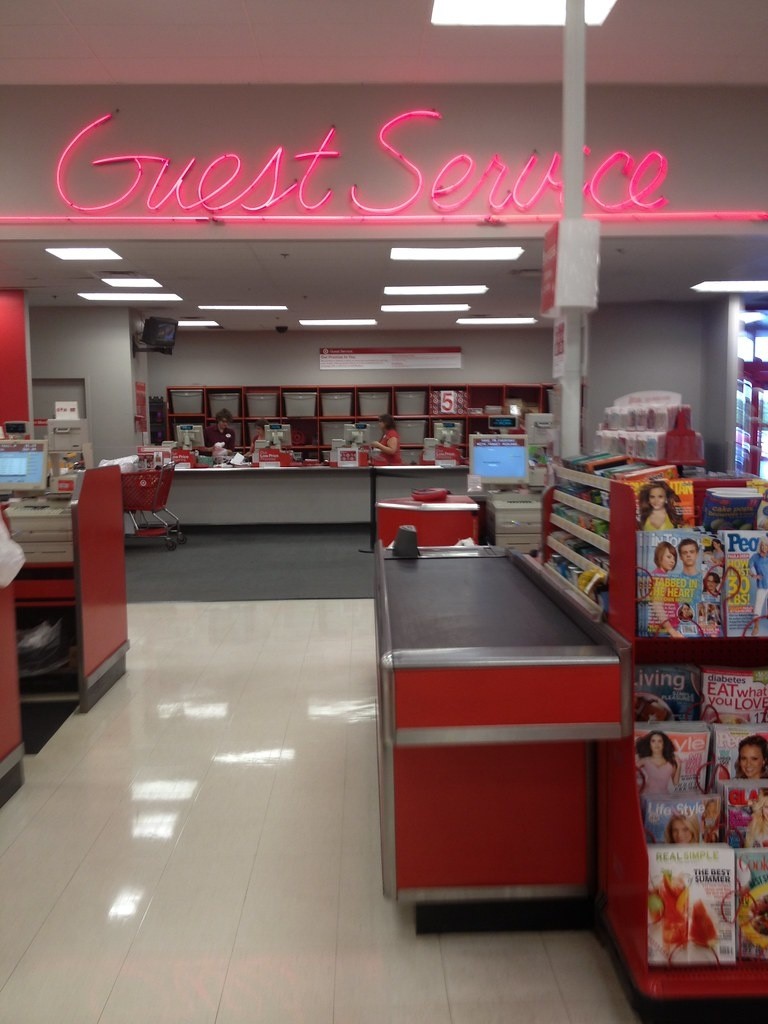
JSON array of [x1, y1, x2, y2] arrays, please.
[[141, 317, 178, 346], [525, 413, 553, 446], [434, 422, 461, 444], [344, 424, 370, 445], [177, 425, 204, 447], [469, 434, 529, 485], [48, 419, 87, 452], [0, 439, 48, 489], [265, 424, 292, 445]]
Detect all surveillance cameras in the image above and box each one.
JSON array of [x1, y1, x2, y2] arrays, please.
[[275, 326, 288, 333]]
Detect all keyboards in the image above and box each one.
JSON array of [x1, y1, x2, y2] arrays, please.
[[4, 506, 71, 515], [492, 500, 541, 508]]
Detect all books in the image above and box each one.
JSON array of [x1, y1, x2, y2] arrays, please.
[[635, 478, 768, 968]]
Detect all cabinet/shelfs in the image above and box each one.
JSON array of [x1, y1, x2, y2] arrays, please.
[[165, 384, 586, 461]]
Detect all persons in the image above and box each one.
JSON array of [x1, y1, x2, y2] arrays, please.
[[202, 408, 236, 456], [678, 603, 692, 619], [747, 537, 768, 635], [665, 810, 700, 844], [638, 480, 689, 532], [701, 798, 720, 843], [710, 538, 725, 566], [249, 419, 269, 454], [702, 572, 722, 596], [678, 538, 700, 575], [746, 537, 768, 636], [651, 541, 677, 573], [634, 730, 681, 794], [369, 414, 403, 466], [735, 734, 768, 781]]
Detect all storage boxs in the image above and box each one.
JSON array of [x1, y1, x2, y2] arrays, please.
[[247, 392, 278, 416], [321, 422, 352, 446], [212, 422, 242, 446], [321, 392, 352, 416], [209, 392, 240, 416], [170, 389, 204, 412], [358, 392, 390, 416], [394, 420, 427, 444], [247, 422, 278, 448], [360, 421, 382, 443], [284, 392, 316, 417], [395, 392, 426, 415]]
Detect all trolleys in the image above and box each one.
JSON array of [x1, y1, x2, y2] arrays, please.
[[122, 457, 187, 551]]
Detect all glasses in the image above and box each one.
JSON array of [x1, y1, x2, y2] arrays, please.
[[222, 420, 228, 423]]
